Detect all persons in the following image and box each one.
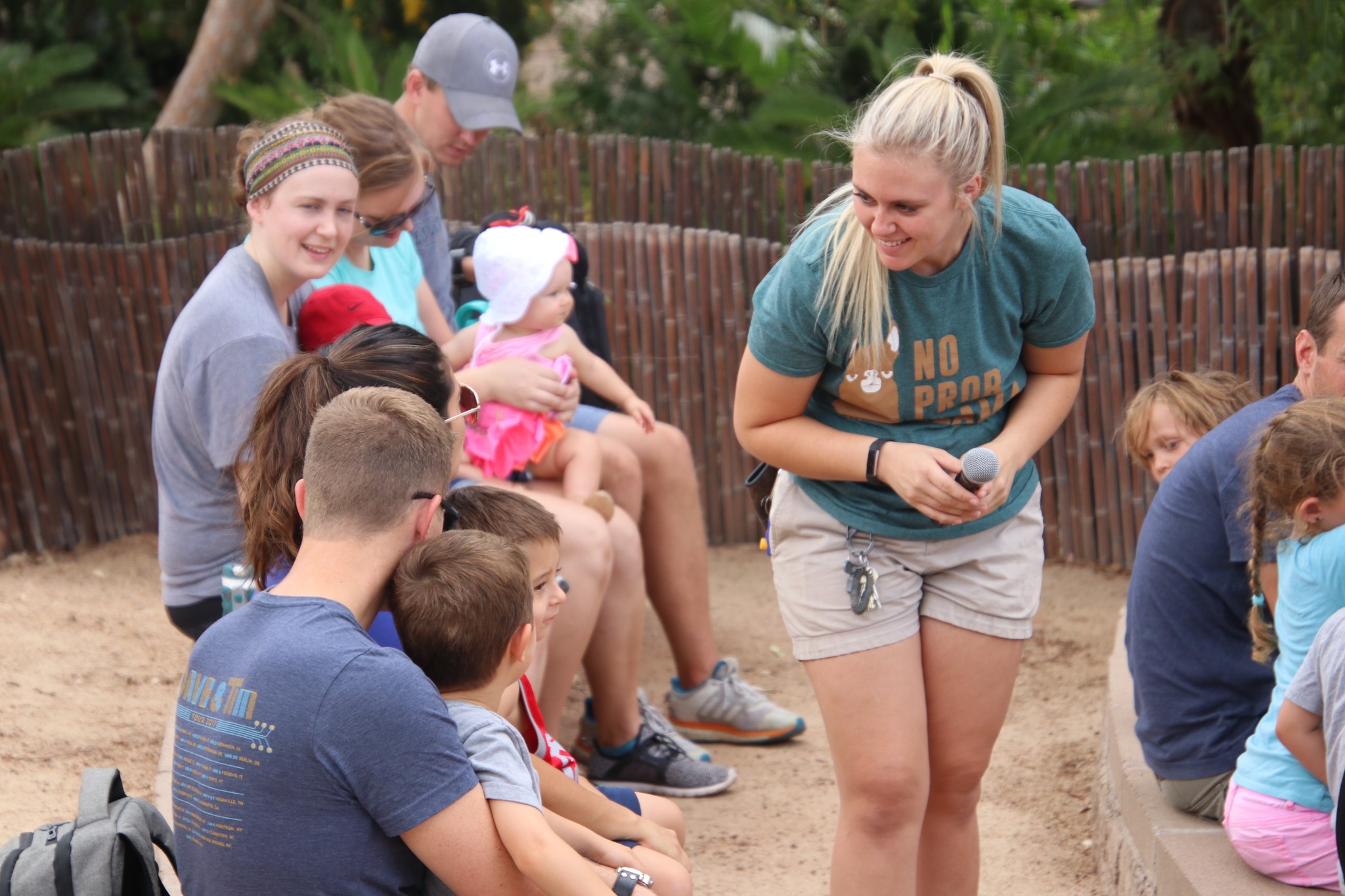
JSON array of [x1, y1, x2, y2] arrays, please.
[[1222, 394, 1345, 890], [1117, 366, 1261, 486], [1125, 273, 1345, 815], [152, 9, 809, 896], [733, 48, 1094, 896], [1276, 605, 1345, 896]]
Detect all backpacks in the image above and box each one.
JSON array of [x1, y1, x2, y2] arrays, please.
[[0, 767, 179, 896]]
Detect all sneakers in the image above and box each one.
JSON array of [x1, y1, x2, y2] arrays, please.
[[570, 689, 711, 764], [664, 659, 806, 744], [586, 723, 737, 798]]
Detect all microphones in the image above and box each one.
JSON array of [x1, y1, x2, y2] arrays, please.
[[931, 446, 1001, 528]]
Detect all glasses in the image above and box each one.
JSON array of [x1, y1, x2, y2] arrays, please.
[[411, 492, 461, 532], [444, 382, 481, 425], [354, 179, 436, 236]]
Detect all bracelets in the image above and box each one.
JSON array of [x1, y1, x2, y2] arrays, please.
[[866, 439, 895, 488]]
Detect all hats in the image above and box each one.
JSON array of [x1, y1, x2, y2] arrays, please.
[[411, 12, 524, 138], [472, 226, 578, 326], [298, 283, 393, 353]]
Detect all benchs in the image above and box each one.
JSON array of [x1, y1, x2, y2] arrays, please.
[[1094, 607, 1345, 896]]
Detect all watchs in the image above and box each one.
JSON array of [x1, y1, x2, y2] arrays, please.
[[611, 867, 655, 896]]
